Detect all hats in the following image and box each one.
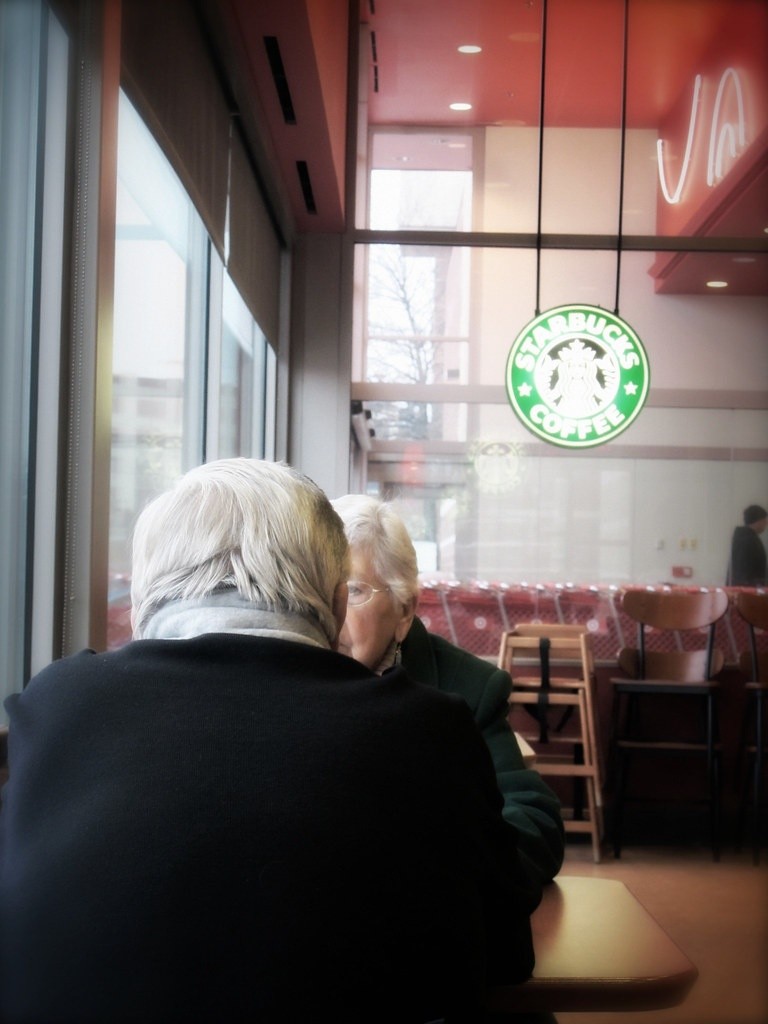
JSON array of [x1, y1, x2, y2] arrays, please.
[[743, 505, 767, 524]]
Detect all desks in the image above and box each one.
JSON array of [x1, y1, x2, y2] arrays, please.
[[476, 655, 621, 671], [531, 876, 694, 1008]]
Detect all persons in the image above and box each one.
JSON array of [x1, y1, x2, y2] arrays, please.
[[0, 457, 565, 1023], [725, 505, 768, 587]]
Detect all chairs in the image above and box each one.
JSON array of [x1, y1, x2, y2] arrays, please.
[[600, 582, 731, 863], [498, 619, 606, 863], [733, 591, 767, 864]]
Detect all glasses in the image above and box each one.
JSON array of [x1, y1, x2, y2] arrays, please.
[[348, 582, 390, 607]]
